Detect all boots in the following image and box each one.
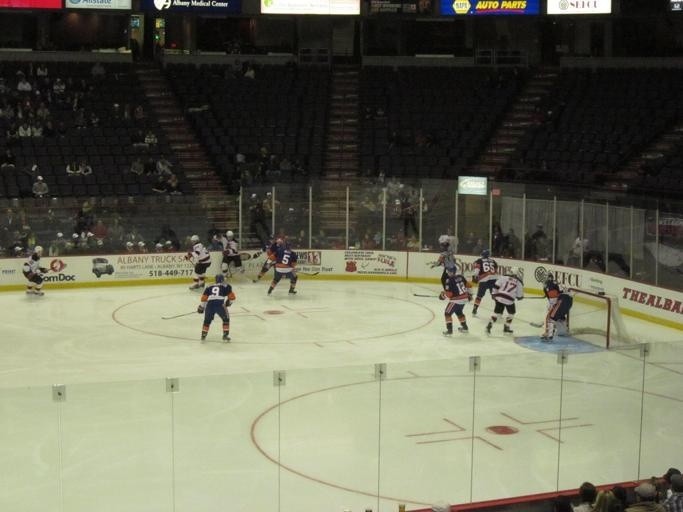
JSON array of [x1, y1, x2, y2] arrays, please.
[[441, 310, 515, 336], [188, 282, 205, 289], [252, 275, 299, 295], [202, 330, 232, 340], [25, 288, 45, 295]]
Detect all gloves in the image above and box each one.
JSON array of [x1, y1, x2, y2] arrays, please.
[[197, 305, 204, 314], [439, 291, 447, 300]]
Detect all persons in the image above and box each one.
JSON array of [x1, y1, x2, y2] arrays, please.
[[0, 48, 589, 343], [553, 468, 683, 512]]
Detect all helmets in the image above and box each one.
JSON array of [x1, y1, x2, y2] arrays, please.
[[215, 273, 225, 283], [480, 249, 490, 258], [226, 230, 234, 237], [446, 265, 457, 273], [190, 235, 199, 241], [33, 245, 44, 252]]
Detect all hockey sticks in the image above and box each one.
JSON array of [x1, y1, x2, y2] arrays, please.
[[162, 311, 198, 319], [410, 289, 439, 298], [530, 322, 544, 327], [295, 271, 319, 276], [522, 294, 547, 299], [39, 259, 60, 270]]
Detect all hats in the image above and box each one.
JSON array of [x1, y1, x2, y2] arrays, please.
[[56, 229, 96, 238], [14, 245, 23, 254], [123, 240, 173, 250]]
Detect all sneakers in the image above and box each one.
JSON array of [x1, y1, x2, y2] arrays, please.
[[539, 334, 553, 340]]
